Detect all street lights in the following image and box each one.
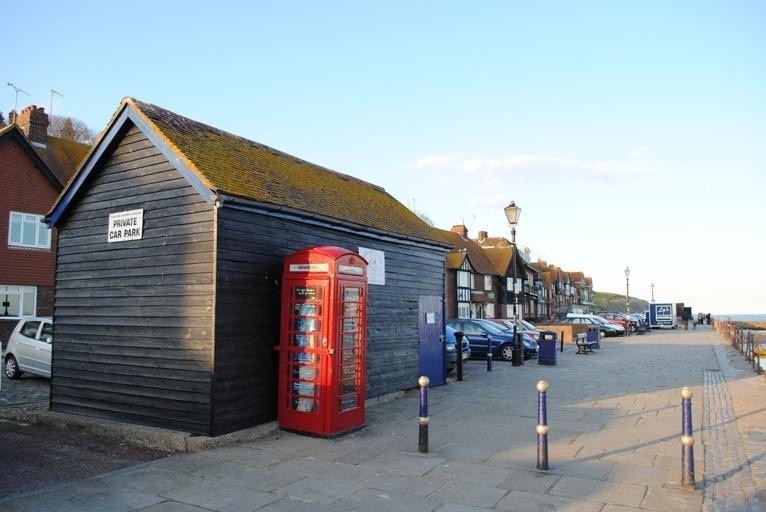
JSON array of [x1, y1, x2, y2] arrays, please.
[[503, 199, 524, 365], [650, 282, 656, 303], [624, 264, 631, 336]]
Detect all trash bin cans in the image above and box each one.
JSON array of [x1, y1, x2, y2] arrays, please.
[[587, 324, 601, 349], [538, 330, 558, 365]]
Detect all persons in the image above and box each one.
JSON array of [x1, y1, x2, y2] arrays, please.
[[691, 312, 712, 331]]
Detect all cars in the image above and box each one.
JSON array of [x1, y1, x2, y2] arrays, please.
[[1, 315, 52, 380], [556, 310, 646, 337], [445, 317, 537, 361], [445, 323, 471, 371], [470, 317, 546, 348]]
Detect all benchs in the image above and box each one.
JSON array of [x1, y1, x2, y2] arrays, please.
[[575, 331, 596, 354]]
